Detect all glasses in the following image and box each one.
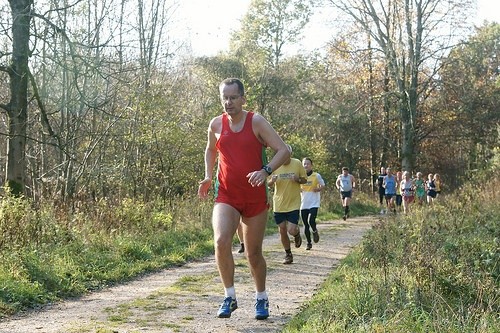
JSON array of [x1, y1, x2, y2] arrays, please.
[[222, 95, 241, 103]]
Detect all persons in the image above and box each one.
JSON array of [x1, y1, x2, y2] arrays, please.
[[266, 143, 307, 265], [335, 168, 356, 221], [197, 79, 291, 320], [234, 219, 245, 256], [377, 167, 441, 217], [298, 157, 325, 250]]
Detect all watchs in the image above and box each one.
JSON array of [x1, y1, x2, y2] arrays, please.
[[261, 165, 273, 175]]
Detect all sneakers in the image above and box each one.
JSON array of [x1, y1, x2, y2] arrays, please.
[[238, 243, 245, 253], [294, 225, 302, 248], [306, 242, 312, 250], [312, 229, 319, 243], [254, 299, 269, 319], [217, 296, 238, 318], [283, 253, 293, 264], [342, 210, 351, 221]]
